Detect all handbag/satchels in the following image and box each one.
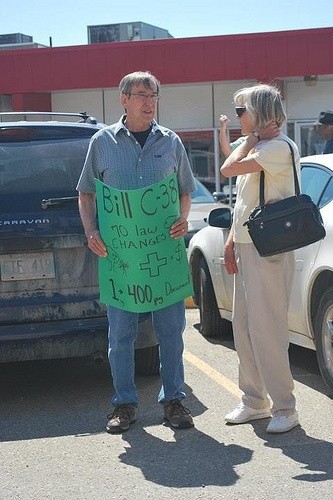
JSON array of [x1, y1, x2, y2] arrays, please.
[[243, 194, 327, 257]]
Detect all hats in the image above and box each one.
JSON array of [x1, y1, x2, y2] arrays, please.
[[313, 110, 333, 126]]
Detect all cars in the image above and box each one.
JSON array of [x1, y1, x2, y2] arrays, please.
[[186, 151, 333, 397], [183, 176, 239, 249]]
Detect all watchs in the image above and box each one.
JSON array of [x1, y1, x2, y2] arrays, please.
[[251, 131, 260, 142]]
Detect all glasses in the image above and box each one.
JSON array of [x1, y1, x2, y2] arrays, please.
[[127, 93, 161, 103], [235, 106, 246, 117]]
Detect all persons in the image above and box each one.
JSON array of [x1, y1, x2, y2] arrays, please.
[[312, 110, 333, 154], [220, 114, 249, 158], [220, 84, 301, 433], [76, 71, 195, 433]]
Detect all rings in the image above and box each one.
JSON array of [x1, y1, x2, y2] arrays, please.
[[182, 229, 184, 232]]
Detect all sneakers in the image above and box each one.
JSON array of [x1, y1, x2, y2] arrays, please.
[[266, 412, 300, 433], [224, 401, 271, 424], [164, 398, 194, 428], [106, 403, 137, 432]]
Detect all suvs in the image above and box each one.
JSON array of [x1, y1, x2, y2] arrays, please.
[[0, 109, 161, 377]]
